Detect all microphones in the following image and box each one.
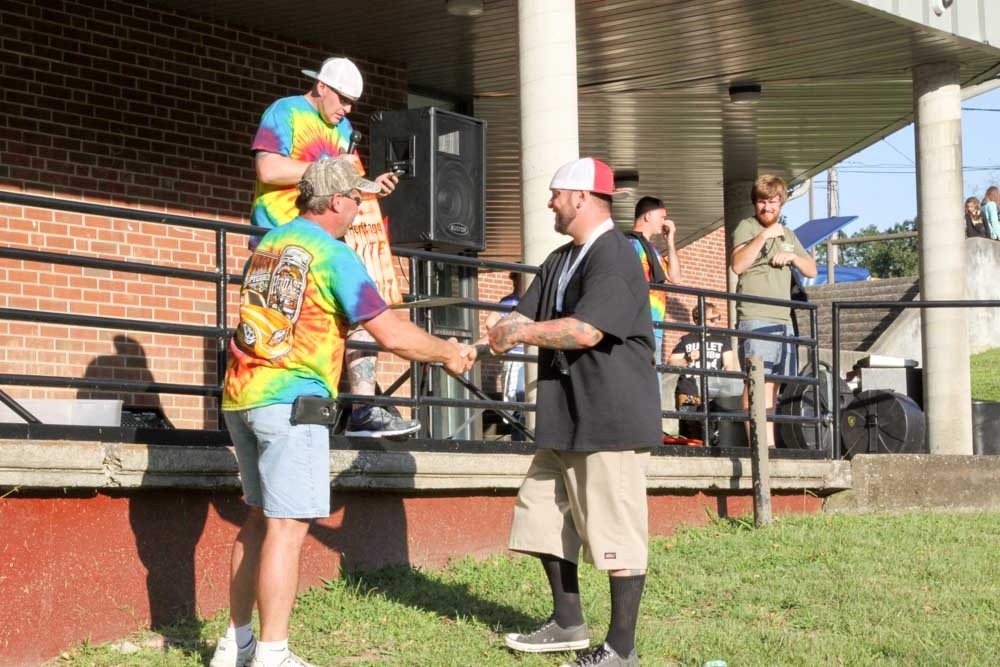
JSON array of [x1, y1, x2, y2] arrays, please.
[[347, 131, 362, 155]]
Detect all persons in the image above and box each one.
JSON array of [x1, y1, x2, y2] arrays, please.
[[624, 194, 681, 439], [205, 156, 481, 667], [727, 174, 818, 449], [963, 186, 1000, 241], [667, 302, 737, 409], [484, 261, 526, 443], [458, 158, 664, 667], [248, 56, 423, 438]]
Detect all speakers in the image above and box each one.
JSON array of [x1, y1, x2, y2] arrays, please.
[[367, 107, 486, 252]]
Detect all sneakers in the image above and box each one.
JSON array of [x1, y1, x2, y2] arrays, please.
[[210, 637, 256, 667], [345, 406, 422, 437], [560, 642, 640, 667], [251, 643, 314, 667], [502, 619, 590, 652]]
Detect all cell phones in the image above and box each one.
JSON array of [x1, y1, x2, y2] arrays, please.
[[292, 397, 337, 425], [389, 169, 405, 179]]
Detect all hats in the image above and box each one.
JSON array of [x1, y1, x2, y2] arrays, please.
[[301, 156, 381, 197], [550, 157, 629, 200], [300, 55, 363, 103]]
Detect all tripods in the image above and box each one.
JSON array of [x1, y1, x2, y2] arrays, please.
[[383, 249, 535, 442]]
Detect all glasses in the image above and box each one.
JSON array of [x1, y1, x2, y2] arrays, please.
[[343, 193, 360, 206], [705, 314, 722, 322], [332, 89, 354, 105]]
